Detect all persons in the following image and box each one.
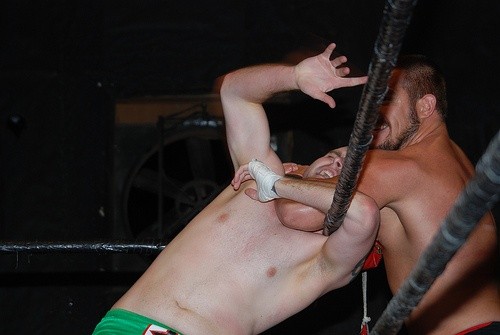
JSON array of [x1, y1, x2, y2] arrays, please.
[[91, 43, 380, 335], [279, 50, 500, 335]]
